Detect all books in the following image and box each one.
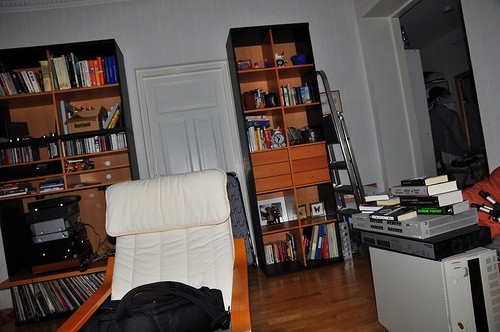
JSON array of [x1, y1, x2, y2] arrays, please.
[[245, 83, 340, 265], [359, 175, 471, 221], [0, 53, 128, 321]]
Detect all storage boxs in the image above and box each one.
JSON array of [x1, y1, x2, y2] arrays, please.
[[66, 160, 94, 173], [64, 105, 106, 135], [0, 122, 28, 140], [247, 115, 268, 130]]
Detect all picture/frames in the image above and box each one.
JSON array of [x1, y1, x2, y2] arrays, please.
[[299, 204, 307, 222], [310, 202, 326, 218], [455, 69, 482, 150], [258, 195, 288, 226], [237, 60, 252, 72]]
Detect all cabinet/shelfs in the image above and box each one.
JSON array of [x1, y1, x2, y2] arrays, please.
[[0, 38, 142, 328], [225, 22, 345, 278]]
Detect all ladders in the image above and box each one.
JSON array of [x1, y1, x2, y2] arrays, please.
[[316, 69, 368, 254]]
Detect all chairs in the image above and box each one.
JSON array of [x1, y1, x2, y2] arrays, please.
[[56, 168, 254, 332], [462, 167, 500, 244]]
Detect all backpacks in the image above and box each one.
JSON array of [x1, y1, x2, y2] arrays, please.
[[85, 281, 231, 332]]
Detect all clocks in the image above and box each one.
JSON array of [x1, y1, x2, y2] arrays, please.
[[274, 134, 285, 144]]
